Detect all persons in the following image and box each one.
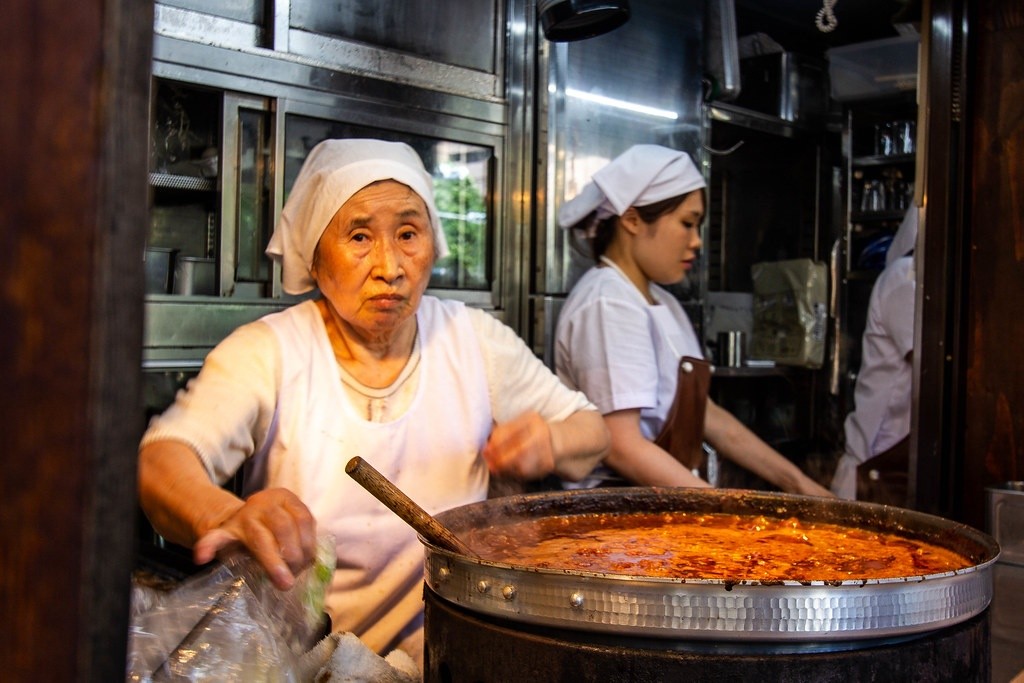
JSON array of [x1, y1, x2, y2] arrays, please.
[[819, 192, 919, 509], [139, 136, 611, 683], [549, 143, 838, 500]]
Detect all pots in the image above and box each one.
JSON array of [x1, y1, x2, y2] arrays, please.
[[403, 485, 1004, 642]]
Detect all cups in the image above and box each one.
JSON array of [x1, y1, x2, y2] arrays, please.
[[717, 330, 745, 367]]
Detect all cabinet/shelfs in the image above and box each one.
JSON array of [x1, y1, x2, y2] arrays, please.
[[843, 87, 919, 507], [698, 98, 828, 492], [144, 1, 519, 370]]
